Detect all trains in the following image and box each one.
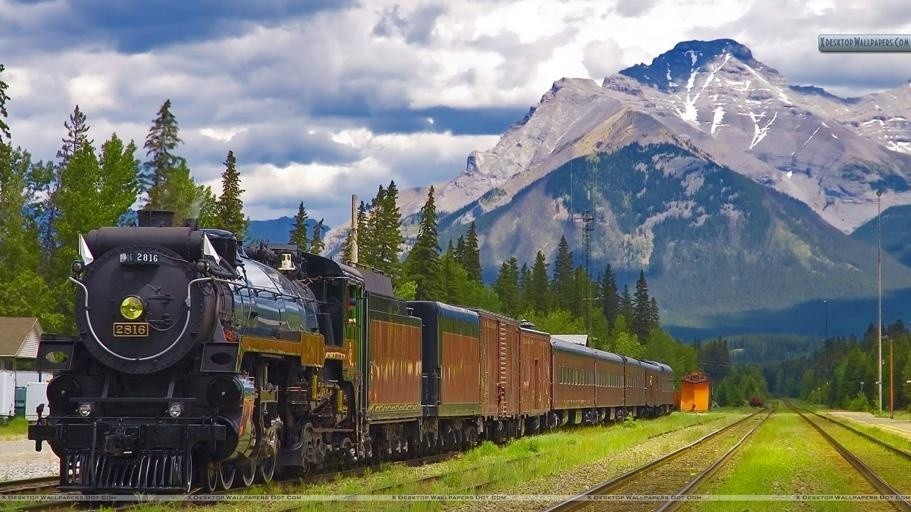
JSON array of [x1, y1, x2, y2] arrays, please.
[[25, 209, 674, 497]]
